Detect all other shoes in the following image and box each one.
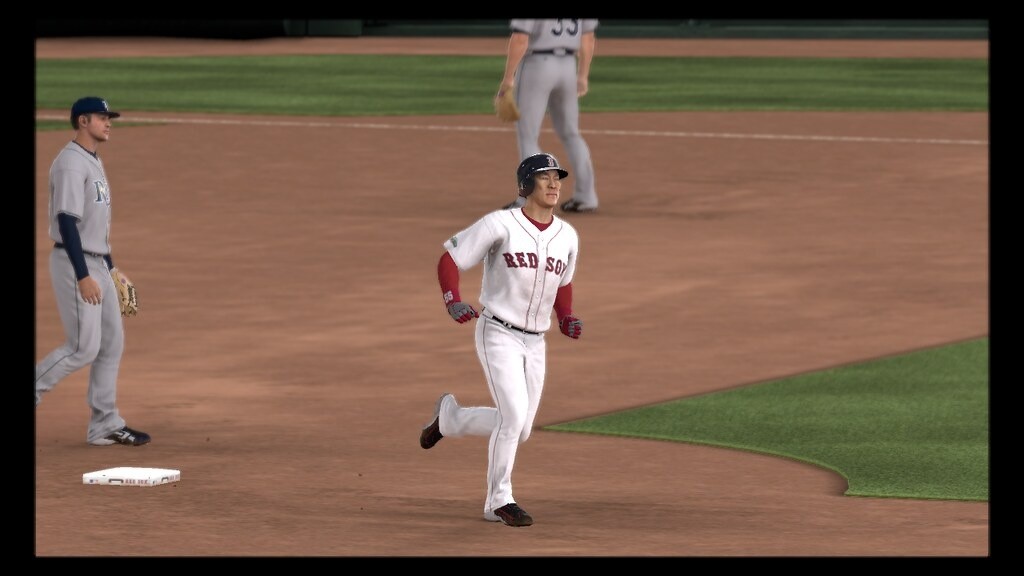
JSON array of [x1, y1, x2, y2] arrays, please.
[[560, 200, 592, 211], [504, 201, 520, 209]]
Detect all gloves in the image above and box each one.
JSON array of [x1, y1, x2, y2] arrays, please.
[[448, 301, 479, 323], [560, 315, 581, 339]]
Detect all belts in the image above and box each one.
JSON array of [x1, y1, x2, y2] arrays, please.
[[532, 49, 573, 55], [482, 307, 538, 334], [53, 242, 102, 256]]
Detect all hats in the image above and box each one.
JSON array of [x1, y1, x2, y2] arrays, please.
[[71, 97, 120, 119]]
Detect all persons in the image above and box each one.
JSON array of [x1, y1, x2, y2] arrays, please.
[[34, 96, 151, 447], [497, 19, 598, 213], [421, 152, 582, 526]]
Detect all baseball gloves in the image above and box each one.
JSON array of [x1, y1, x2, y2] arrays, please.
[[109, 266, 138, 318], [494, 82, 521, 122]]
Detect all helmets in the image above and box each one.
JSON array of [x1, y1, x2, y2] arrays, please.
[[517, 154, 568, 197]]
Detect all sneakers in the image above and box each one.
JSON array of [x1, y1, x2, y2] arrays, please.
[[89, 427, 150, 446], [484, 504, 532, 526], [419, 394, 449, 449]]
